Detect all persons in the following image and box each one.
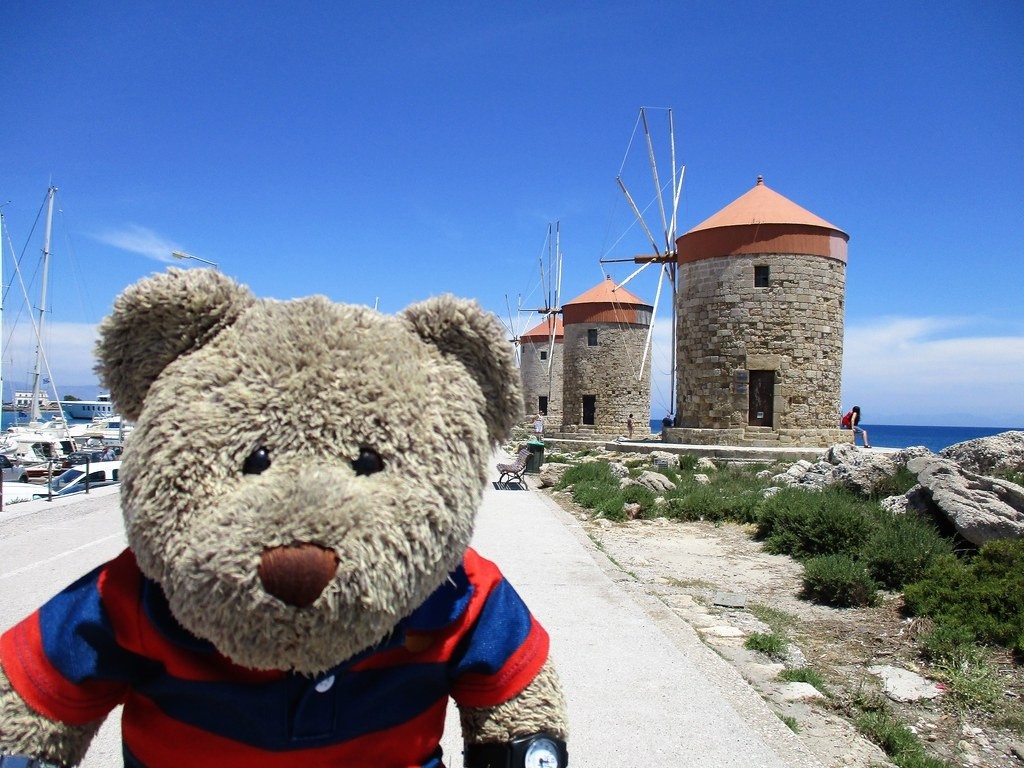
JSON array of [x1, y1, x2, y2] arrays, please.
[[661, 413, 676, 443], [536, 410, 544, 441], [628, 414, 636, 439], [848, 406, 873, 448]]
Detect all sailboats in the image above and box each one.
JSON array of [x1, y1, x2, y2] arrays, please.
[[2, 174, 137, 468]]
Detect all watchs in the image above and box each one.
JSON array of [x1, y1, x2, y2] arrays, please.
[[462, 732, 568, 768]]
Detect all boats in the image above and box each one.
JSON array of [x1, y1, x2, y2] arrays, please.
[[59, 392, 120, 420], [2, 390, 60, 412]]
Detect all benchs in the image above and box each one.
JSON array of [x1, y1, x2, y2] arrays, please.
[[497, 449, 533, 492]]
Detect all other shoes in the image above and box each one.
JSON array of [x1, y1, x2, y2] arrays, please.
[[864, 445, 872, 448]]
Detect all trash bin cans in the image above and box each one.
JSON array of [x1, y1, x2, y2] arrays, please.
[[526, 442, 545, 473]]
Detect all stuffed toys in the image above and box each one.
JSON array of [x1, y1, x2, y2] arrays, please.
[[0, 266, 571, 768]]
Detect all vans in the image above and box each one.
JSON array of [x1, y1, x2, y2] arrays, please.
[[61, 448, 116, 467]]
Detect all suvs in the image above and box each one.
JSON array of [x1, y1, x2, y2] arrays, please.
[[30, 460, 123, 499], [0, 455, 29, 484]]
